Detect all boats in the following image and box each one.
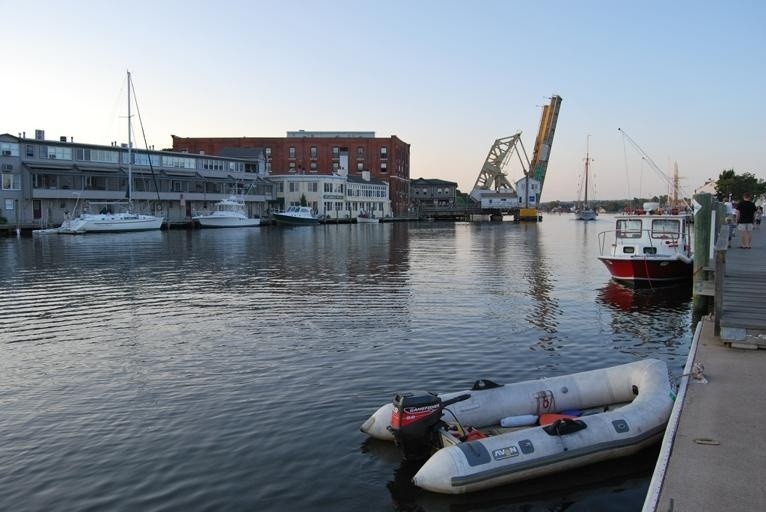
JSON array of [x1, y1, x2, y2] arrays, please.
[[360, 359, 675, 495], [597, 201, 694, 290]]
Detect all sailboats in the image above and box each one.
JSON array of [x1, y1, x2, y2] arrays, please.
[[574, 134, 596, 220]]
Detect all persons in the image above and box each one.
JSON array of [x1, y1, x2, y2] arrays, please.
[[723, 191, 763, 249], [99, 207, 107, 214]]
